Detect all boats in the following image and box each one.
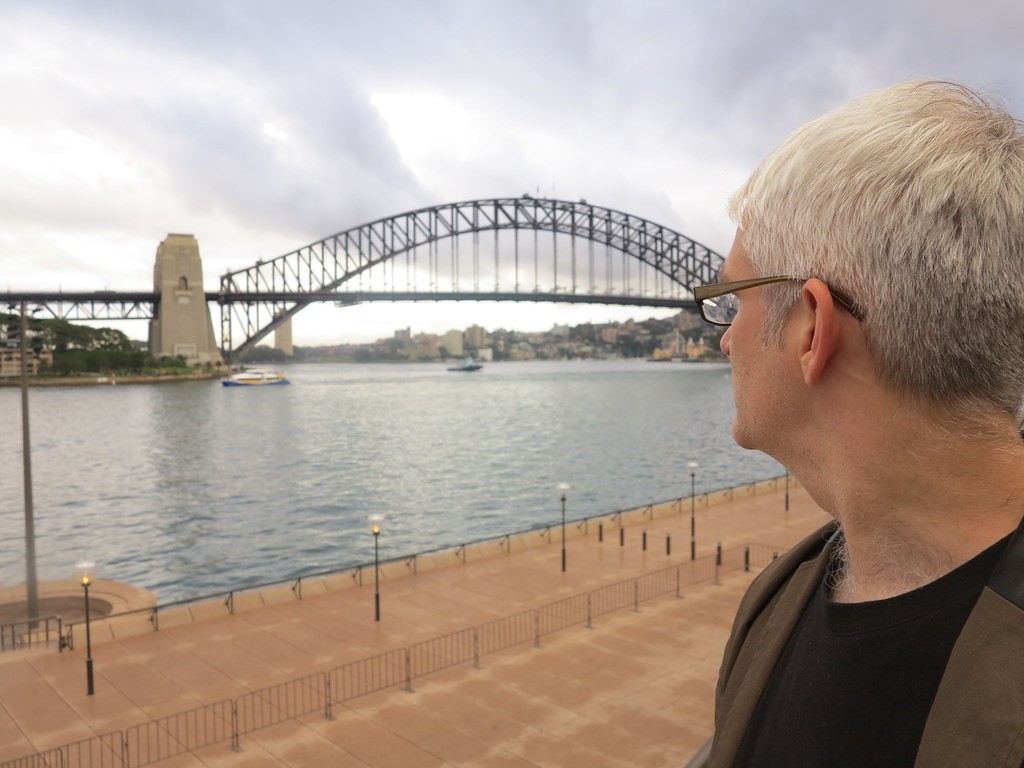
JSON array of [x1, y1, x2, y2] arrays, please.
[[447, 356, 483, 372], [222, 369, 290, 388]]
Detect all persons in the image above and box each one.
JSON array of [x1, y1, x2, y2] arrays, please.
[[693, 80, 1024, 768]]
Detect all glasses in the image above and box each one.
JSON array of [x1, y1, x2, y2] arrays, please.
[[693, 275, 868, 324]]
